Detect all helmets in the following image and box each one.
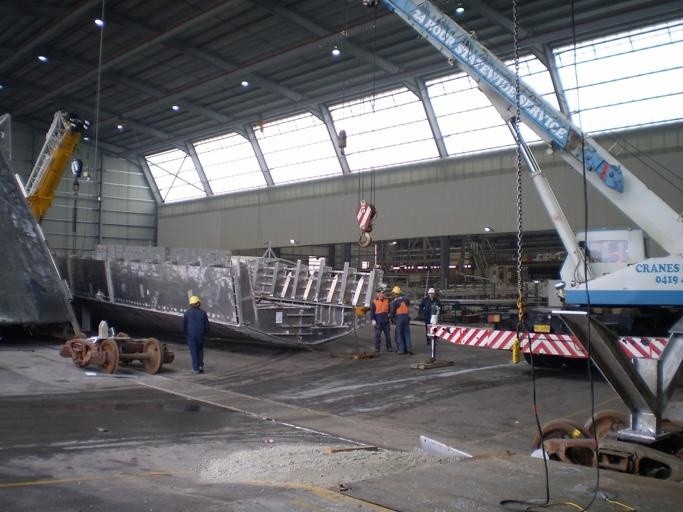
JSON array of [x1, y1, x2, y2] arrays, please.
[[392, 287, 401, 293], [189, 296, 201, 305], [375, 287, 385, 292], [428, 288, 436, 294]]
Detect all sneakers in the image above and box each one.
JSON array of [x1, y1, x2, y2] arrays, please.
[[193, 366, 203, 374]]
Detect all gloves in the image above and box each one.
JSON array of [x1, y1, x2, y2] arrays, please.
[[372, 320, 376, 326]]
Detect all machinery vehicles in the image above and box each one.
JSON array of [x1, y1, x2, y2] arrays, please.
[[22, 109, 90, 223], [354, 2, 682, 483]]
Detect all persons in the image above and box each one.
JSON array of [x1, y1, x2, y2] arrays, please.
[[418, 287, 442, 345], [370, 287, 393, 353], [388, 286, 415, 356], [181, 295, 209, 374]]
